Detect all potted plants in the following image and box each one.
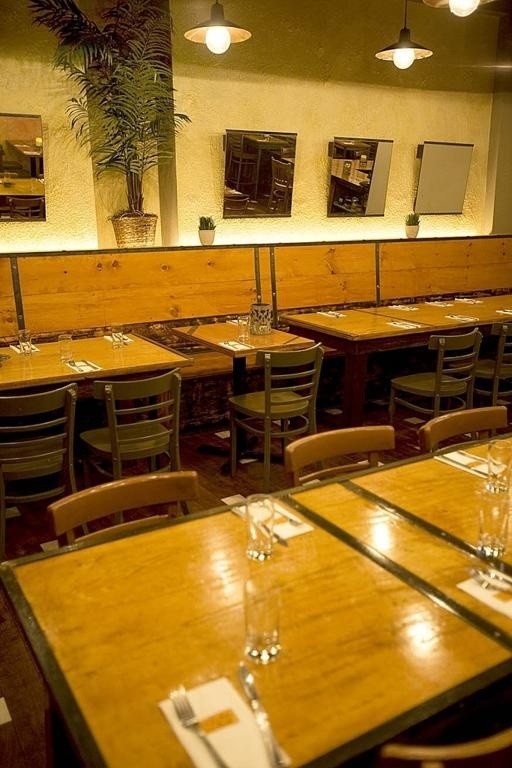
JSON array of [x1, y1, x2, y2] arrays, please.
[[28, 0, 192, 249], [404, 214, 420, 238], [198, 217, 216, 245]]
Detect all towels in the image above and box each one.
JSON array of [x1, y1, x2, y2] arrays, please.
[[156, 677, 293, 768], [319, 298, 512, 321], [456, 570, 512, 620]]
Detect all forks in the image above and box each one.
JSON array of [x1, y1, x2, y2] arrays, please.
[[275, 506, 304, 530], [65, 358, 84, 374], [168, 687, 230, 767], [472, 570, 510, 596]]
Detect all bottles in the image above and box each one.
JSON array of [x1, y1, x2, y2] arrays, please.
[[342, 161, 351, 175]]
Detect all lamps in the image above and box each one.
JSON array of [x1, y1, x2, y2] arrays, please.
[[423, 0, 495, 18], [183, 0, 252, 54], [374, 0, 434, 69]]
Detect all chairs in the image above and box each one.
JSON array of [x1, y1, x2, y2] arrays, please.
[[48, 467, 200, 550], [1, 379, 81, 545], [224, 134, 293, 214], [0, 144, 45, 220], [452, 322, 512, 407], [415, 404, 512, 459], [286, 422, 403, 492], [380, 727, 512, 768], [386, 325, 485, 434], [79, 365, 191, 528], [222, 340, 326, 493]]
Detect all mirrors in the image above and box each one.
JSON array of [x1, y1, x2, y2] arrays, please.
[[0, 113, 47, 222]]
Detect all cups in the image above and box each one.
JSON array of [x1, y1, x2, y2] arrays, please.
[[17, 330, 31, 357], [245, 493, 275, 559], [477, 438, 512, 557], [111, 325, 124, 351], [58, 334, 73, 362], [244, 576, 282, 662], [238, 317, 249, 340]]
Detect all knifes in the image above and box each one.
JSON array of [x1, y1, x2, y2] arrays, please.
[[483, 565, 512, 586], [79, 359, 99, 371], [236, 663, 285, 765], [258, 518, 288, 548], [440, 454, 488, 481]]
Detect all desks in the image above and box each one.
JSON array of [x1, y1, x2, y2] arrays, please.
[[170, 316, 315, 474], [0, 321, 196, 394]]
